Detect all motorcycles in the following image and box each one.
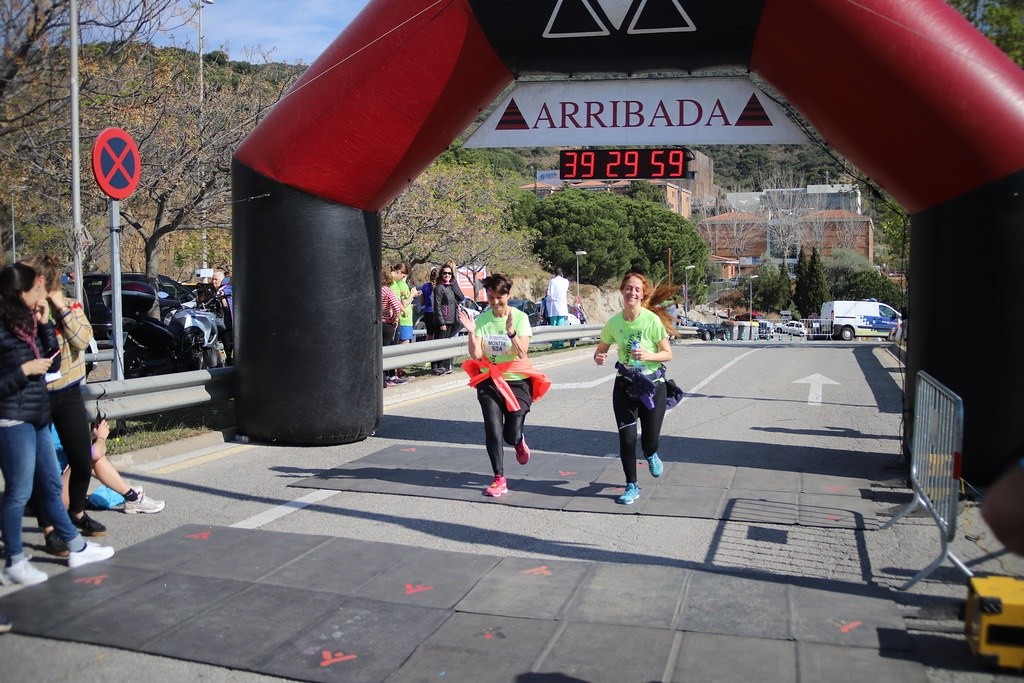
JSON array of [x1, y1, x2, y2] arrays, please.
[[106, 277, 233, 380]]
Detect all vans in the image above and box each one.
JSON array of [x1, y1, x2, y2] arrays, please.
[[821, 298, 902, 341]]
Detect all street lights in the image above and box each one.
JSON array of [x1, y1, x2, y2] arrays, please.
[[686, 265, 695, 326], [574, 251, 587, 309], [749, 275, 759, 340]]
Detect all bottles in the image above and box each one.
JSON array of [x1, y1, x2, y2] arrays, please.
[[630, 340, 642, 368]]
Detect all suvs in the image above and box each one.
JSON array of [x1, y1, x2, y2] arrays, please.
[[688, 322, 714, 341], [703, 324, 727, 341], [77, 271, 197, 340]]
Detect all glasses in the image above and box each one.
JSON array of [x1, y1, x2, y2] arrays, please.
[[442, 271, 453, 276]]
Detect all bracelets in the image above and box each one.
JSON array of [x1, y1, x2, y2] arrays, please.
[[508, 330, 516, 338], [594, 353, 598, 357], [58, 306, 67, 314]]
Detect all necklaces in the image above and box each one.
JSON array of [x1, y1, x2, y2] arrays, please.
[[627, 313, 634, 321]]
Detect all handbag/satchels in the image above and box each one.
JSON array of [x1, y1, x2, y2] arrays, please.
[[88, 484, 125, 511]]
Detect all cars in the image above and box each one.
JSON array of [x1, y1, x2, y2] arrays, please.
[[458, 296, 480, 332], [507, 299, 540, 328], [735, 313, 766, 322], [774, 321, 808, 337], [758, 322, 774, 338]]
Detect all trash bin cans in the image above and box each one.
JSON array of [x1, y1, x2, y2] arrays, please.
[[736, 321, 752, 341], [720, 321, 739, 341], [748, 321, 759, 341]]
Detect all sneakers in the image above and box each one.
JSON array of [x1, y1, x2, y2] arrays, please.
[[68, 542, 116, 568], [129, 486, 145, 496], [513, 434, 530, 465], [124, 498, 165, 514], [68, 510, 106, 537], [0, 556, 48, 586], [485, 475, 508, 496], [385, 372, 414, 387], [617, 482, 640, 505], [44, 531, 71, 555], [645, 452, 663, 477]]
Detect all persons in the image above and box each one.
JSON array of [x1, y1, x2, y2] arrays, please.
[[593, 273, 682, 504], [458, 274, 552, 497], [382, 263, 464, 388], [0, 253, 166, 632], [542, 267, 570, 349], [212, 271, 233, 360]]
